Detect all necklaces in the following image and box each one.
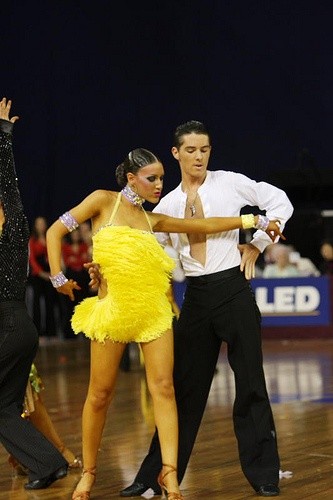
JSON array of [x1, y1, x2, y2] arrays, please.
[[188, 195, 198, 215], [121, 186, 145, 207]]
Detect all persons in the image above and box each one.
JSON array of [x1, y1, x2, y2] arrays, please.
[[83, 120, 295, 500], [6, 364, 83, 477], [26, 215, 92, 344], [45, 147, 285, 500], [253, 239, 333, 279], [0, 98, 71, 491]]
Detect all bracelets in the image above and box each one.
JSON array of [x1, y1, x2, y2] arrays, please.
[[49, 271, 68, 288], [255, 215, 270, 232]]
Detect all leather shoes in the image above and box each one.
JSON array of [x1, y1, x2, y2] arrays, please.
[[256, 484, 279, 496], [24, 466, 68, 490], [120, 480, 162, 496]]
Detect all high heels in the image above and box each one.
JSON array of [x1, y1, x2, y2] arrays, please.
[[157, 463, 184, 500], [55, 445, 83, 469], [72, 466, 97, 500], [7, 454, 31, 479]]
